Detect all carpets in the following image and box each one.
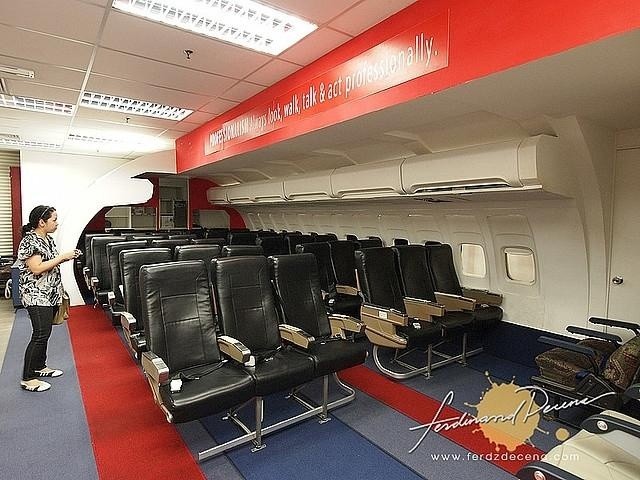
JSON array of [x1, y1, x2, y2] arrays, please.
[[0, 307, 640, 480]]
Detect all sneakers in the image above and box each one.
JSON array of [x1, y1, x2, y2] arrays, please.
[[35, 369, 63, 377], [21, 379, 50, 392]]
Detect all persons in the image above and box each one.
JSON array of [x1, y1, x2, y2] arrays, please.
[[17, 203, 84, 392]]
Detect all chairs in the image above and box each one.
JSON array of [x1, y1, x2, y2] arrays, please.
[[516, 409, 640, 480], [531, 317, 640, 417]]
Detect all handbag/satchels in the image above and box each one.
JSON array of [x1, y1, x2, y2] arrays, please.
[[52, 291, 70, 324]]
